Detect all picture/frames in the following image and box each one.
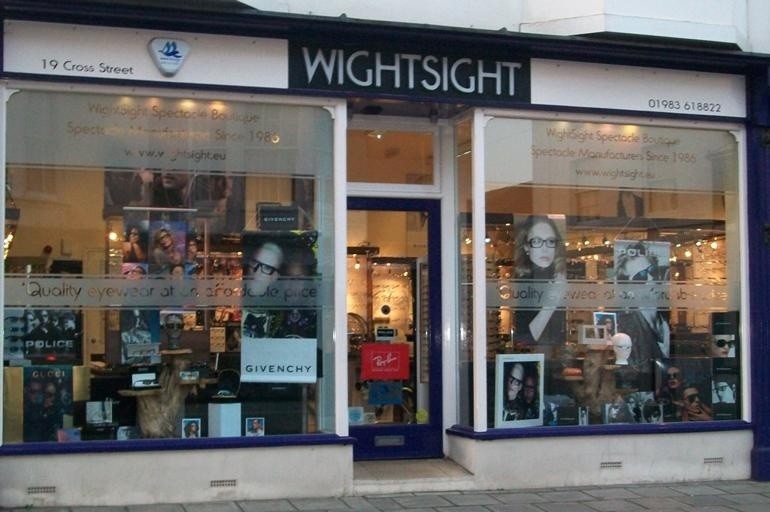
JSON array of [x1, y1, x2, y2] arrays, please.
[[181, 418, 200, 439], [493, 351, 548, 430], [246, 417, 265, 435]]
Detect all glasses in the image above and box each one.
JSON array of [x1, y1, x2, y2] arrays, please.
[[159, 321, 187, 331], [712, 338, 735, 349], [665, 372, 681, 379], [633, 263, 659, 282], [247, 258, 281, 276], [682, 393, 706, 404], [525, 237, 559, 249], [5, 316, 26, 353], [507, 373, 523, 386]]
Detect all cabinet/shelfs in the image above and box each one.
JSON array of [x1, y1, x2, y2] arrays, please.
[[549, 338, 640, 419], [102, 211, 213, 371], [119, 344, 219, 441]]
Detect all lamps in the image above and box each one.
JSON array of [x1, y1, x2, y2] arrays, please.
[[463, 223, 728, 263], [364, 128, 387, 138], [354, 255, 361, 272]]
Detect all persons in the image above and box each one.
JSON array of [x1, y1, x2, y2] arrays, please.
[[614, 240, 670, 388], [548, 400, 561, 425], [605, 366, 713, 423], [243, 237, 287, 301], [514, 213, 566, 344], [503, 362, 524, 422], [712, 376, 738, 420], [522, 374, 537, 422], [104, 134, 243, 370], [4, 259, 83, 442], [577, 407, 589, 425], [713, 336, 734, 357], [184, 420, 200, 439], [611, 332, 632, 367], [280, 243, 318, 309], [282, 308, 311, 339], [248, 418, 265, 437]]
